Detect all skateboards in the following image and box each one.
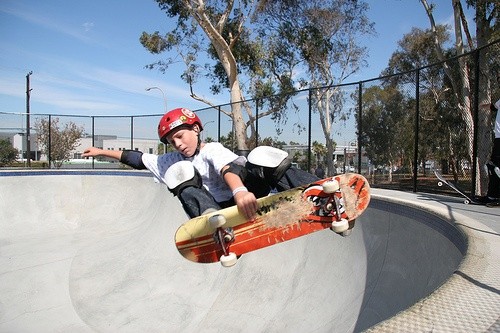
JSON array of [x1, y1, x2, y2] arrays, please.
[[434, 170, 477, 205], [175, 173, 370, 268]]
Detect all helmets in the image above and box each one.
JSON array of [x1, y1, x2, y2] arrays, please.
[[156, 106, 205, 143]]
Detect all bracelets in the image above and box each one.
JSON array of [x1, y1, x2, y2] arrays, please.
[[232, 187, 248, 196]]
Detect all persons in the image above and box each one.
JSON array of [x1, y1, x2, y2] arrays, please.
[[315, 164, 325, 178], [81, 107, 324, 222], [476, 99, 500, 208]]
